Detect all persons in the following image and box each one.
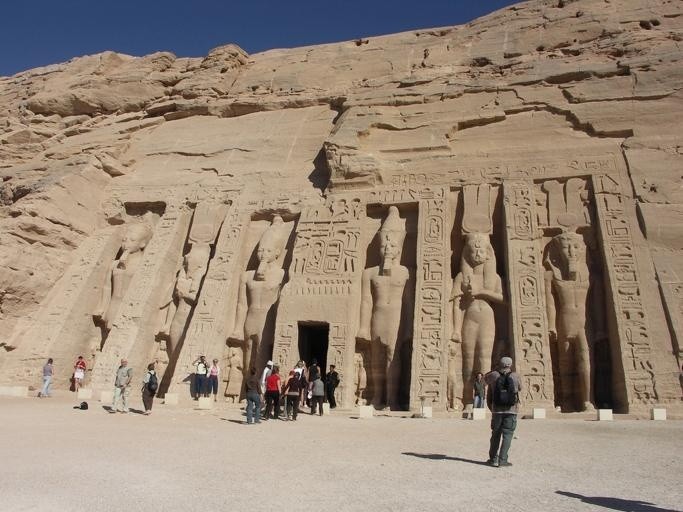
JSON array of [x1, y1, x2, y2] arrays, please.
[[354, 216, 413, 409], [89, 214, 152, 351], [39, 358, 53, 397], [192, 356, 219, 400], [73, 356, 86, 391], [244, 360, 341, 424], [481, 355, 524, 467], [473, 372, 487, 409], [543, 232, 599, 412], [167, 244, 210, 353], [240, 218, 291, 389], [450, 231, 506, 414], [141, 364, 158, 415], [109, 359, 133, 413]]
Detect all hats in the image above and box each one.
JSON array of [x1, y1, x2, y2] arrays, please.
[[499, 357, 512, 367]]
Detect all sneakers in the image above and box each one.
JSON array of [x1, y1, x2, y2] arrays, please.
[[487, 459, 498, 466], [498, 463, 512, 466]]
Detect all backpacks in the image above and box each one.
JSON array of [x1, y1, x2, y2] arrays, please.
[[148, 372, 159, 391], [494, 371, 520, 407]]
